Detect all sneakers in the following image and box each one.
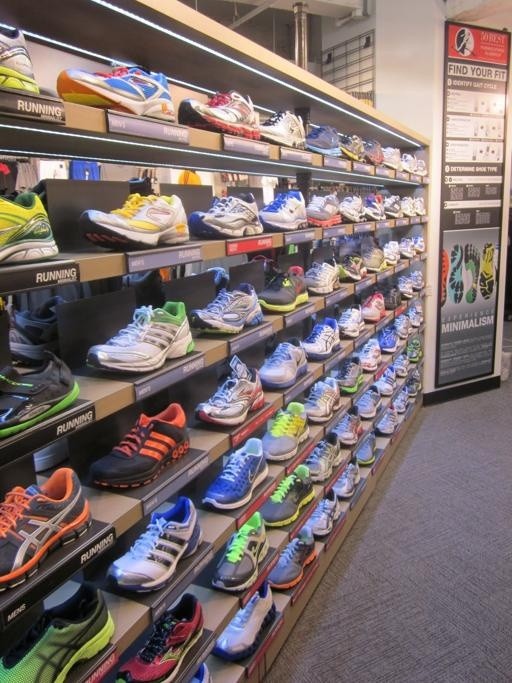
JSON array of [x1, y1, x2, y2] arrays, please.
[[195, 317, 376, 537], [178, 89, 310, 335], [335, 271, 424, 434], [0, 191, 59, 265], [305, 124, 426, 295], [0, 402, 319, 682], [56, 63, 176, 121], [0, 350, 81, 437], [1, 30, 40, 98], [5, 295, 69, 363], [85, 302, 196, 374], [78, 192, 192, 251]]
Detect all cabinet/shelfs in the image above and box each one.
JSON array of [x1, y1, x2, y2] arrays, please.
[[1, 3, 431, 681]]
